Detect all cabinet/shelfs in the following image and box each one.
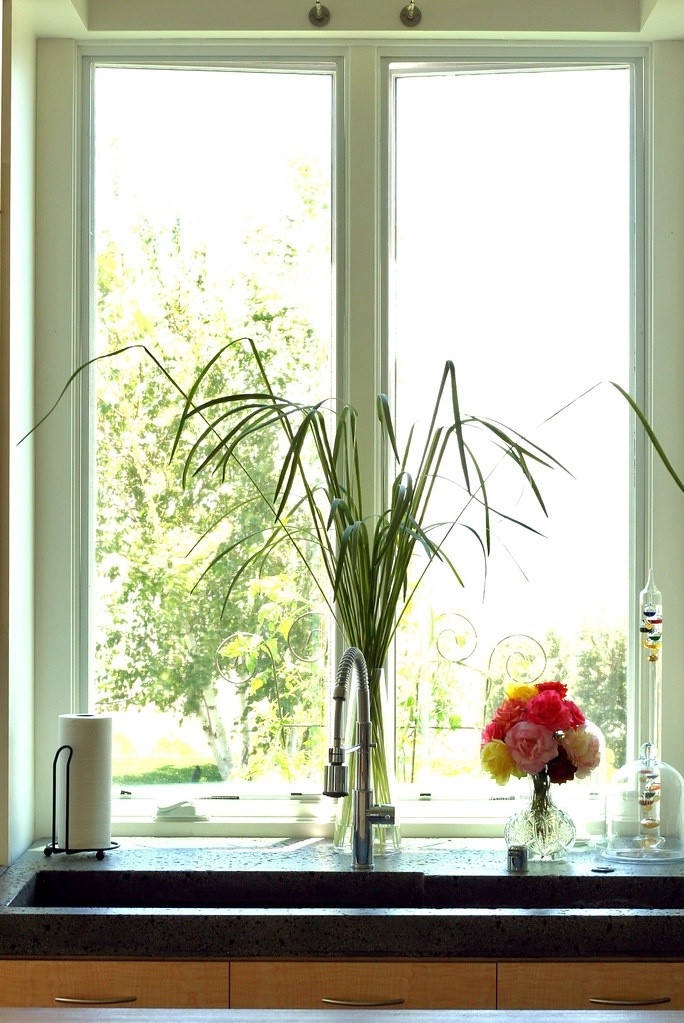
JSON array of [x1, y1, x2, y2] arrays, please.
[[0, 958, 684, 1011]]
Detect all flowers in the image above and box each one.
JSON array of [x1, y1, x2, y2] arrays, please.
[[477, 679, 599, 786]]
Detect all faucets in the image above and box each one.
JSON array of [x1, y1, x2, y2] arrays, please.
[[321, 647, 396, 869]]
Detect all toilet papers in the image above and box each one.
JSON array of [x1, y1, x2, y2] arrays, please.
[[58, 714, 112, 849]]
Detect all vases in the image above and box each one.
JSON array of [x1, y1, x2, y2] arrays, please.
[[332, 667, 401, 855], [503, 790, 577, 856]]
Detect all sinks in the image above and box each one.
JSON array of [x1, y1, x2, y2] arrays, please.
[[5, 870, 683, 912]]
